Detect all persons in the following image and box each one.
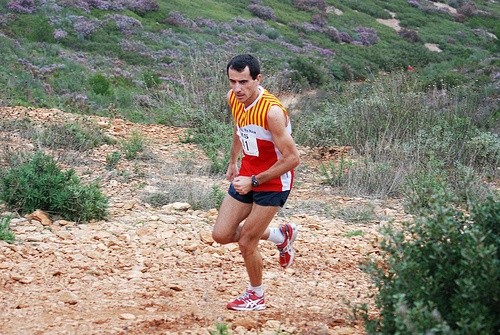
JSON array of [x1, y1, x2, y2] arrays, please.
[[212, 55, 300, 311]]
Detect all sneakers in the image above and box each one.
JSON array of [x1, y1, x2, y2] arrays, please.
[[274, 222, 298, 270], [225, 288, 266, 311]]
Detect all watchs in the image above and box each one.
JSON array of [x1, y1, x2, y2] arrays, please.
[[252, 175, 258, 187]]
